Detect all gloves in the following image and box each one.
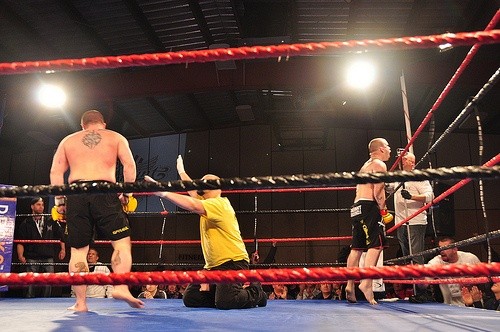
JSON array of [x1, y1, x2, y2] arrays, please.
[[401, 190, 411, 199], [122, 194, 137, 214], [51, 205, 67, 222], [144, 175, 166, 197], [382, 213, 393, 224], [176, 155, 185, 174]]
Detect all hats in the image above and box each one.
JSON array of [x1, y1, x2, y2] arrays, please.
[[29, 197, 44, 205]]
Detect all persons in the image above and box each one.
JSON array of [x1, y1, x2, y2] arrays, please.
[[15, 196, 66, 297], [145, 154, 268, 311], [385, 151, 435, 303], [129, 234, 500, 313], [345, 138, 394, 306], [70, 249, 114, 299], [50, 110, 145, 312]]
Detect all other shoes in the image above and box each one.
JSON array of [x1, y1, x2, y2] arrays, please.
[[251, 281, 268, 307]]
